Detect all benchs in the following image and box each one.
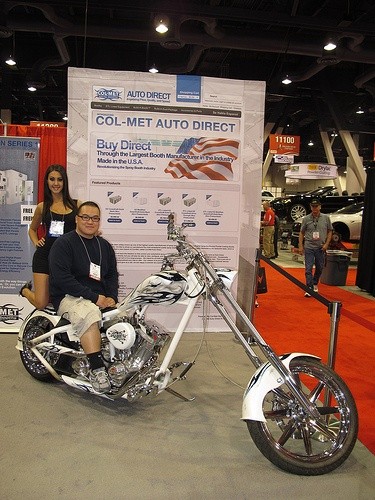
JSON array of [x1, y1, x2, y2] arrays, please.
[[351, 192, 365, 196]]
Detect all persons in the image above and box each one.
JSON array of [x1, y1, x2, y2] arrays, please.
[[48, 202, 119, 392], [299, 199, 333, 296], [261, 201, 280, 259], [20, 165, 101, 310]]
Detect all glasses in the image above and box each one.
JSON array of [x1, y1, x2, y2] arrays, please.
[[76, 214, 100, 222]]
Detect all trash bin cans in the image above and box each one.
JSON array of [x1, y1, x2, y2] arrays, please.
[[321, 248, 353, 286]]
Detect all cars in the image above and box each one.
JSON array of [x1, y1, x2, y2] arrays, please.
[[261, 190, 274, 204], [268, 186, 367, 226], [291, 201, 367, 248]]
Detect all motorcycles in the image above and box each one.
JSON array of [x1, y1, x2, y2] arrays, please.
[[15, 210, 359, 477]]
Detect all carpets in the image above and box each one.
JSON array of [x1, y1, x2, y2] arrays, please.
[[252, 243, 375, 456]]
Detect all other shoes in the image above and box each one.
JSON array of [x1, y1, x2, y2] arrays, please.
[[265, 255, 278, 259]]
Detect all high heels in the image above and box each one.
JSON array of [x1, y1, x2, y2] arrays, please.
[[18, 280, 32, 297]]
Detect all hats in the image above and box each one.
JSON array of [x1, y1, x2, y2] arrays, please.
[[261, 202, 269, 206], [310, 199, 321, 206]]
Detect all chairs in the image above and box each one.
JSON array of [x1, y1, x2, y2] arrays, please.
[[334, 192, 339, 196], [342, 190, 348, 196]]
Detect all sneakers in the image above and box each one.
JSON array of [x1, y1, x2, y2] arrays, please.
[[313, 285, 318, 293], [88, 365, 110, 392], [304, 286, 312, 296]]
